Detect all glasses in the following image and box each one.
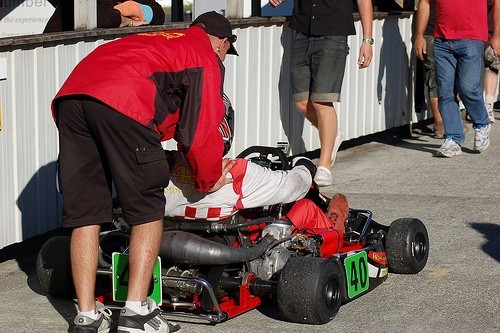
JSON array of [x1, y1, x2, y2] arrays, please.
[[220, 35, 237, 43]]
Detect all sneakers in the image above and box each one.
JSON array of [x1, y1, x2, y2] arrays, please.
[[116, 297, 181, 333], [68, 301, 118, 333], [325, 193, 350, 232], [473, 123, 490, 151], [331, 129, 345, 167], [313, 167, 333, 185], [436, 139, 462, 157]]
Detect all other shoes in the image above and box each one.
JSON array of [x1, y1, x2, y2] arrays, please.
[[484, 103, 495, 122], [433, 120, 445, 138]]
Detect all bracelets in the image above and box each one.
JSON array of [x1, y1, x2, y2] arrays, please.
[[362, 38, 374, 45]]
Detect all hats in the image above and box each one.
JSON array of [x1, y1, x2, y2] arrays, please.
[[190, 11, 239, 56]]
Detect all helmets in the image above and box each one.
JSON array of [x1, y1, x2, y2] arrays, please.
[[218, 92, 234, 158]]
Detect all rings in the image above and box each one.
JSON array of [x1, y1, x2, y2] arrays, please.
[[230, 178, 232, 182]]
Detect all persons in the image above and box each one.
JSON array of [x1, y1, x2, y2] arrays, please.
[[268, 0, 373, 186], [163, 91, 349, 247], [50, 9, 240, 333], [44, 0, 165, 33], [414, 0, 500, 158]]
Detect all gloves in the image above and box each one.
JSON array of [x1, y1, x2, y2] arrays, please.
[[289, 157, 317, 179]]
